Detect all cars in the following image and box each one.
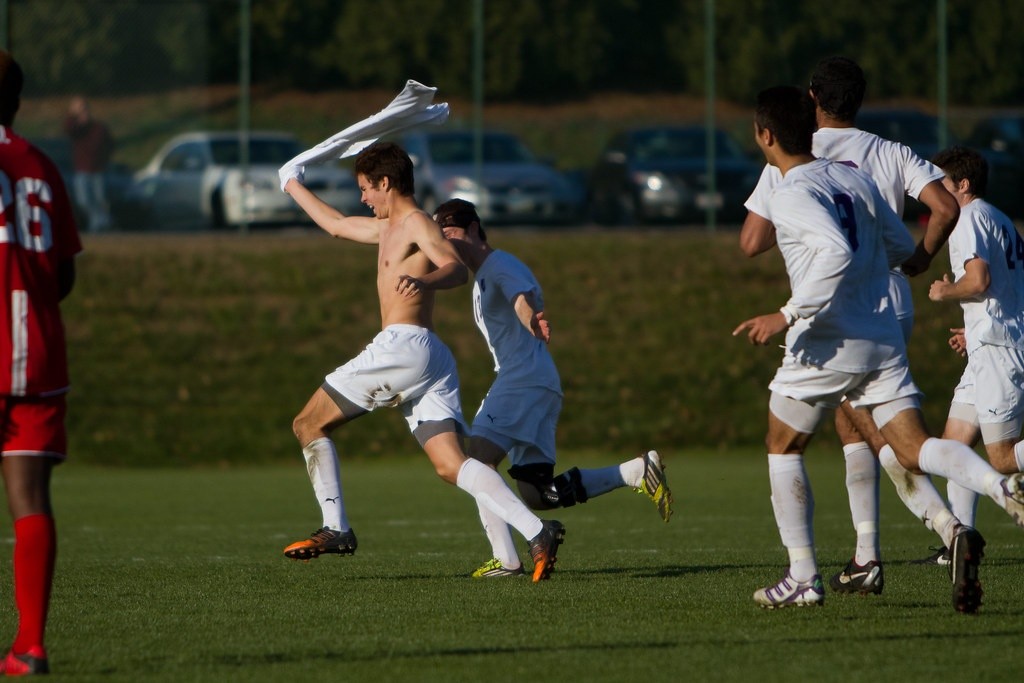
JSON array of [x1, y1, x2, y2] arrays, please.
[[122, 128, 368, 232], [859, 107, 969, 188], [35, 139, 121, 233], [381, 129, 583, 231], [588, 123, 763, 230], [958, 113, 1024, 222]]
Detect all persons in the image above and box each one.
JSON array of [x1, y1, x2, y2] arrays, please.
[[733, 87, 1024, 609], [739, 57, 987, 615], [62, 96, 112, 232], [924, 145, 1024, 565], [284, 141, 566, 582], [0, 50, 85, 677], [433, 198, 673, 577]]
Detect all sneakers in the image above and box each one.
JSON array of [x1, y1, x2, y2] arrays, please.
[[633, 450, 674, 522], [753, 569, 825, 607], [527, 519, 566, 583], [946, 523, 986, 615], [829, 556, 884, 595], [467, 557, 527, 577], [284, 526, 358, 563], [0, 646, 49, 676], [919, 545, 952, 566], [999, 473, 1024, 527]]
